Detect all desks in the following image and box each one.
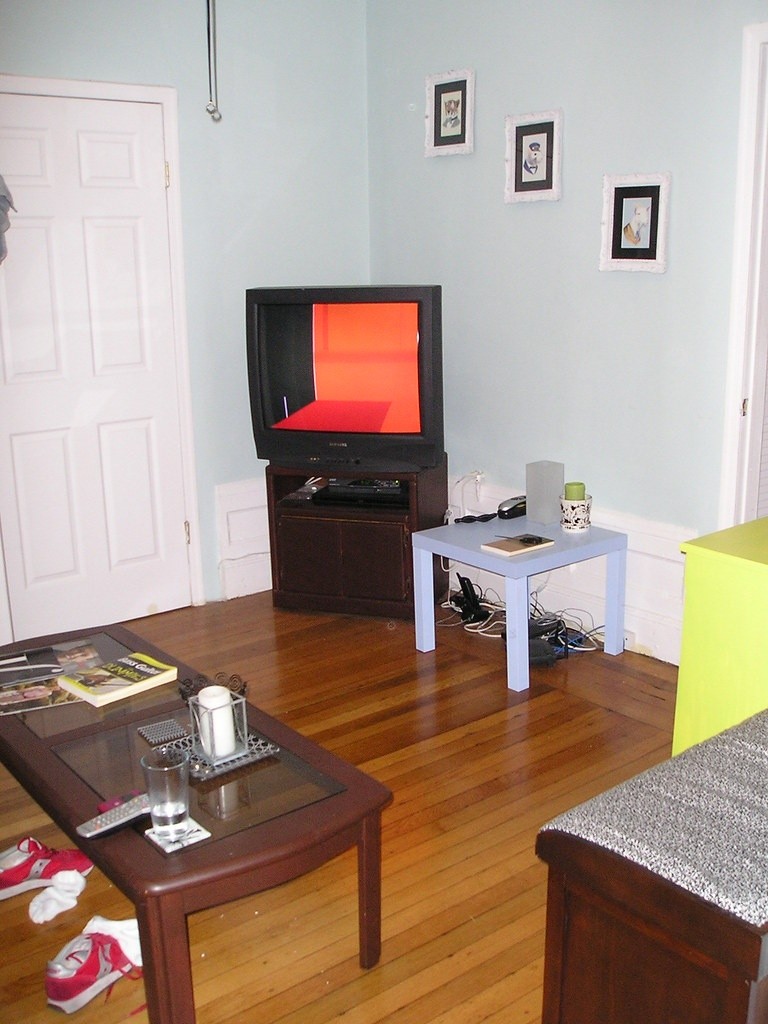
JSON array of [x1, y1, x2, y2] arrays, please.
[[411, 514, 628, 691]]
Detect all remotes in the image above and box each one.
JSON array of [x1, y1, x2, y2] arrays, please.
[[76, 794, 151, 840], [498, 495, 526, 512]]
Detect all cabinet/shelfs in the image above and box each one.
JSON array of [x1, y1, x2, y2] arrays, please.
[[672, 518, 768, 756], [265, 453, 449, 619], [535, 707, 768, 1024]]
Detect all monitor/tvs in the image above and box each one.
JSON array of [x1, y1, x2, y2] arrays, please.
[[243, 285, 445, 470]]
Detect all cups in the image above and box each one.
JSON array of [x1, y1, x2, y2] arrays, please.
[[140, 748, 190, 838]]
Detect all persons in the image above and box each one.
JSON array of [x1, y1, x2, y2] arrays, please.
[[53, 644, 97, 666], [0, 686, 60, 705], [79, 674, 116, 688]]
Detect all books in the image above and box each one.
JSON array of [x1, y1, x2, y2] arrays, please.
[[481, 533, 555, 557], [0, 638, 177, 715]]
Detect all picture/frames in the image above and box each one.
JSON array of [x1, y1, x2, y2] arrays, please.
[[599, 174, 671, 274], [424, 67, 475, 157], [504, 108, 561, 204]]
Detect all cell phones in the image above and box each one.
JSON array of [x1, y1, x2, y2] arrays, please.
[[98, 791, 139, 814]]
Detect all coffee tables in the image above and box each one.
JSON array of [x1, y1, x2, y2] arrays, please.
[[0, 624, 395, 1024]]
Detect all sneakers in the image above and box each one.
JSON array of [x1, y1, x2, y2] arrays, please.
[[0, 835, 94, 901], [45, 933, 147, 1015]]
